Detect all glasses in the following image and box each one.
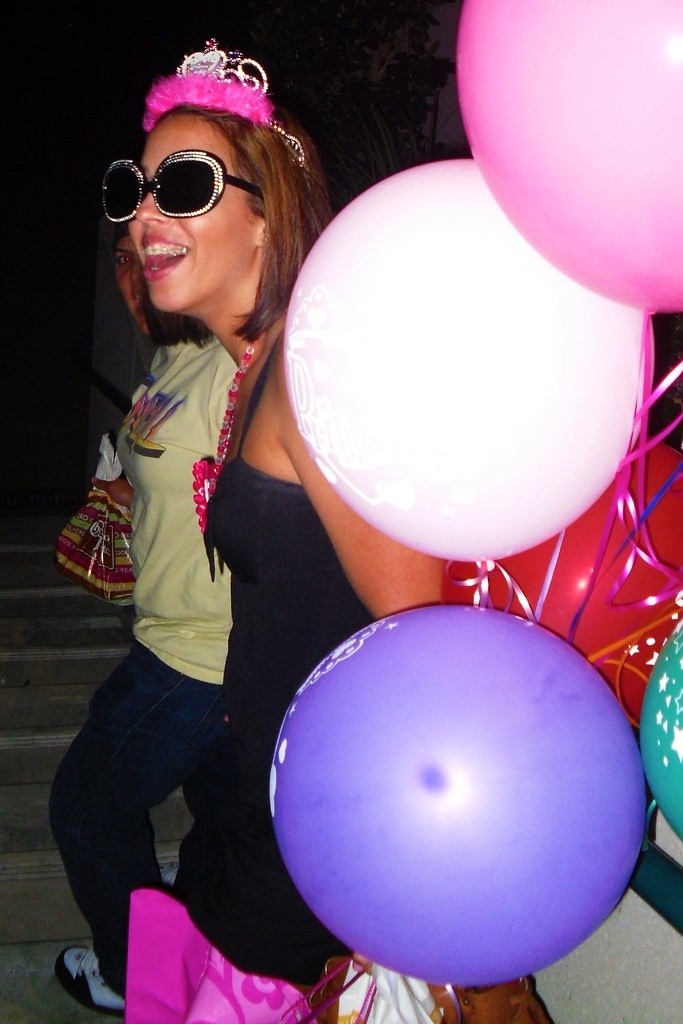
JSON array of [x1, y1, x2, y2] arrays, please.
[[102, 150, 263, 223]]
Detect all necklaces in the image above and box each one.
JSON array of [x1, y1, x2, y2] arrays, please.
[[193, 337, 261, 533]]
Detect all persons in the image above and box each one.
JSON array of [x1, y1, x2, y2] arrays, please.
[[48, 40, 443, 1015]]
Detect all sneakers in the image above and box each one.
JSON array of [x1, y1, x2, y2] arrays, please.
[[56, 944, 125, 1018]]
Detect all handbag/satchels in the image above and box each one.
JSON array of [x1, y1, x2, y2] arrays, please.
[[125, 889, 555, 1024], [55, 433, 137, 606]]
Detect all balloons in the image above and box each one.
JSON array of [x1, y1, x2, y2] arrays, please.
[[447, 438, 683, 720], [641, 614, 683, 848], [269, 601, 643, 987], [456, 0, 683, 310], [282, 160, 654, 561]]
